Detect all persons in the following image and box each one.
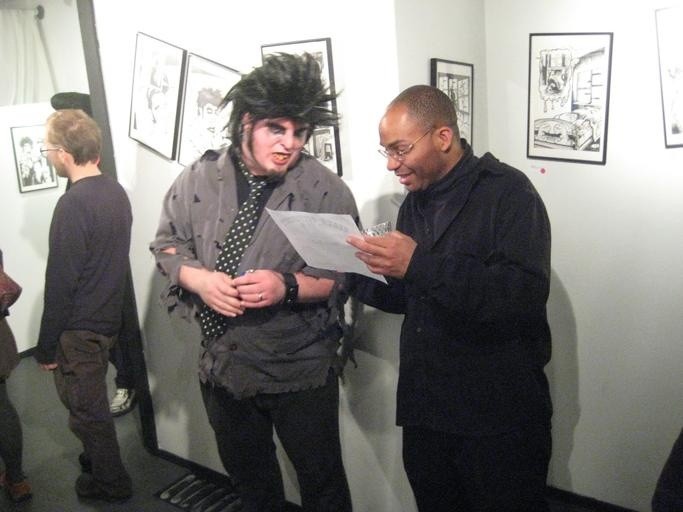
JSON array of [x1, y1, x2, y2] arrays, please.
[[51, 91, 136, 416], [33, 109, 133, 503], [16, 134, 37, 185], [150, 52, 367, 511], [343, 85, 555, 511], [195, 88, 224, 152], [1, 250, 33, 501]]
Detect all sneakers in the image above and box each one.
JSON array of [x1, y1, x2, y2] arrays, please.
[[74, 473, 134, 505], [78, 451, 105, 474], [0, 480, 33, 512]]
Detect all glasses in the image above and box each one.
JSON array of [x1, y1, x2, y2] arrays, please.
[[39, 146, 59, 156], [377, 127, 433, 160]]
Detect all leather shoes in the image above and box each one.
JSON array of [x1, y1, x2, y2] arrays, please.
[[109, 387, 136, 417]]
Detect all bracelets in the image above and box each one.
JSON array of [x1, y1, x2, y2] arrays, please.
[[282, 273, 301, 306]]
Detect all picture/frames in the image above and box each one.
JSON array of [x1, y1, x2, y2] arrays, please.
[[430, 58, 472, 150], [9, 125, 58, 193], [525, 32, 615, 165], [126, 29, 187, 162], [176, 49, 244, 170], [260, 36, 342, 178], [653, 6, 683, 150]]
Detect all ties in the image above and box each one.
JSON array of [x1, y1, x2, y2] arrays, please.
[[198, 146, 272, 342]]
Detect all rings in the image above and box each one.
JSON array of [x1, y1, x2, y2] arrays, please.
[[258, 293, 264, 302]]
